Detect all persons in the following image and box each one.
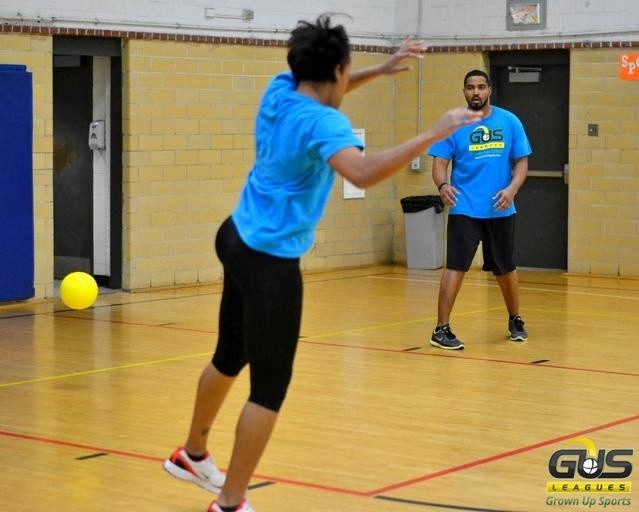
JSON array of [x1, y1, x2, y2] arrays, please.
[[423, 70, 533, 349], [163, 9, 486, 512]]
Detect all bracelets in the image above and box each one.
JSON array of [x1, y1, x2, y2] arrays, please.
[[438, 182, 448, 192]]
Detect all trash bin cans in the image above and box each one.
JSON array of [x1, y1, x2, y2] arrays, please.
[[401, 195, 444, 270]]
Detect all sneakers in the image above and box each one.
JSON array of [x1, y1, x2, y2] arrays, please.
[[507, 315, 528, 341], [163, 446, 260, 512], [430, 324, 465, 350]]
